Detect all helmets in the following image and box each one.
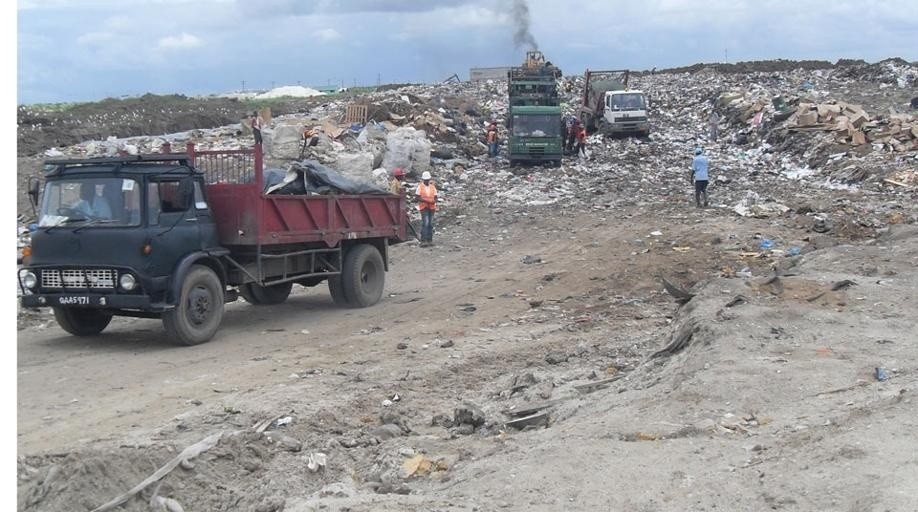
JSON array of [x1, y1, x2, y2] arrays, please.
[[422, 171, 431, 180], [573, 117, 584, 128], [394, 168, 406, 176], [490, 119, 496, 131]]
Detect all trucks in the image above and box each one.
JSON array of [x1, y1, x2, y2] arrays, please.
[[507, 66, 563, 168], [17, 118, 408, 348], [579, 67, 650, 137]]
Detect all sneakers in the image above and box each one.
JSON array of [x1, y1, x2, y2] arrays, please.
[[420, 242, 435, 247]]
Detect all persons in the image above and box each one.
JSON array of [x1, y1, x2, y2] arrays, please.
[[302, 130, 319, 147], [486, 121, 499, 158], [390, 167, 406, 194], [251, 111, 262, 147], [652, 66, 657, 76], [562, 115, 590, 160], [708, 110, 719, 141], [690, 147, 709, 208], [415, 170, 440, 247], [69, 183, 112, 221]]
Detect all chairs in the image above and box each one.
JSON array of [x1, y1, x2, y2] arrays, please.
[[103, 182, 124, 220]]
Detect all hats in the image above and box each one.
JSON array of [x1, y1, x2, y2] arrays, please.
[[695, 148, 701, 154]]
